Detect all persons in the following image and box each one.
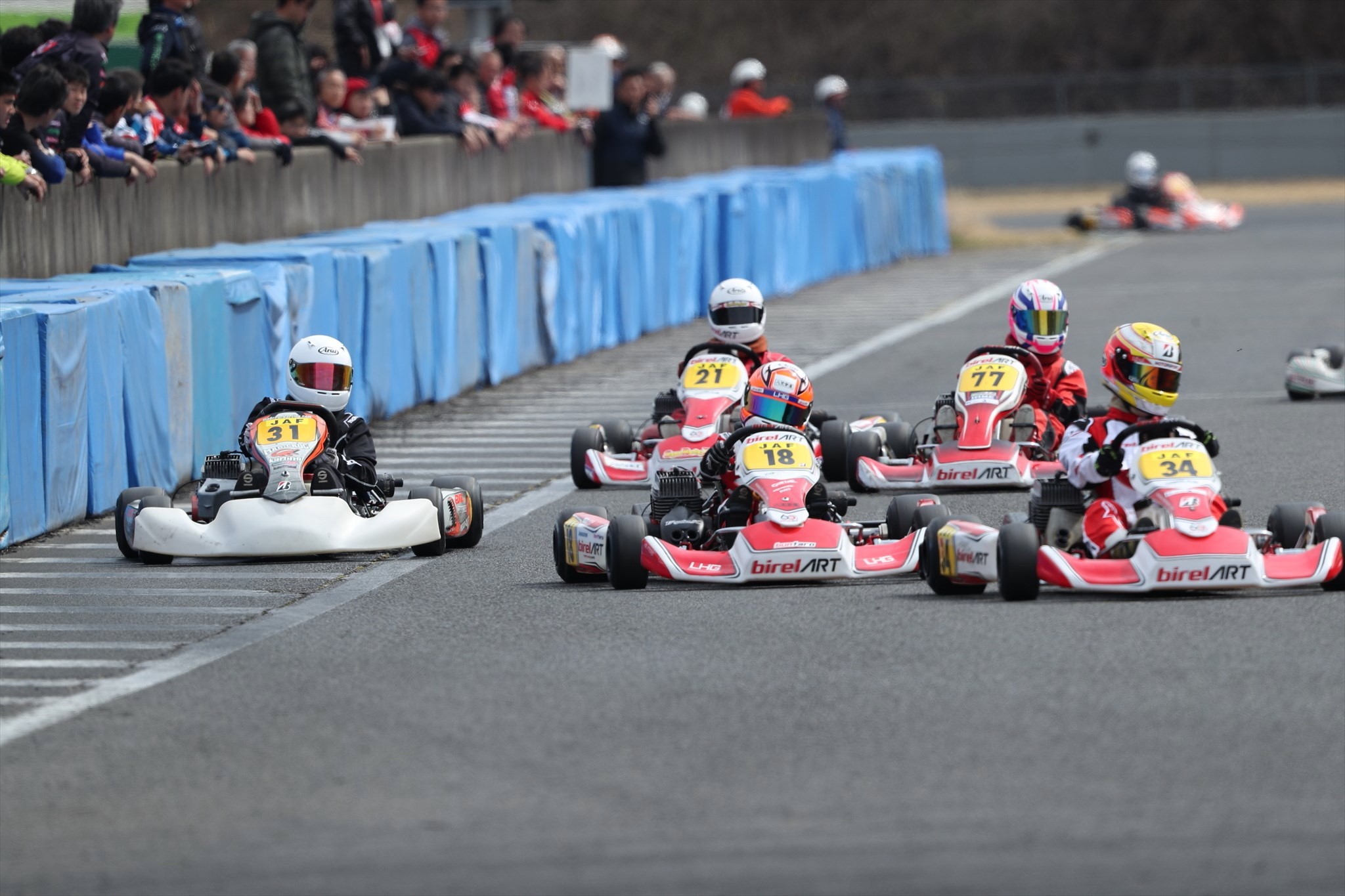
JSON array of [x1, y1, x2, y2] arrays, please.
[[1057, 322, 1242, 560], [722, 58, 791, 118], [1115, 149, 1177, 230], [659, 278, 793, 440], [234, 335, 378, 506], [935, 279, 1087, 460], [0, 0, 710, 201], [699, 361, 831, 550], [814, 75, 852, 154]]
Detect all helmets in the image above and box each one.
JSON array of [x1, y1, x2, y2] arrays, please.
[[1123, 150, 1161, 189], [677, 93, 710, 121], [738, 361, 814, 431], [813, 75, 849, 102], [286, 334, 354, 411], [1100, 322, 1183, 416], [727, 57, 766, 88], [1008, 278, 1068, 355], [708, 277, 766, 345]]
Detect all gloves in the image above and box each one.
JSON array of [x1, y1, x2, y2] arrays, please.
[[1195, 430, 1220, 458], [1095, 444, 1125, 476], [1026, 375, 1051, 400], [314, 447, 340, 472], [244, 422, 253, 457]]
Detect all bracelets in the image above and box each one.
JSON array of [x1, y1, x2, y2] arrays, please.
[[26, 167, 40, 175]]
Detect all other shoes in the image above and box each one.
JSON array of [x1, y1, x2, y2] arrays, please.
[[661, 415, 681, 438], [936, 404, 958, 443], [233, 471, 260, 499], [805, 483, 829, 519], [310, 469, 348, 503], [725, 485, 752, 549], [1133, 518, 1154, 534], [1011, 404, 1036, 449], [1219, 510, 1242, 529], [734, 421, 744, 431]]
